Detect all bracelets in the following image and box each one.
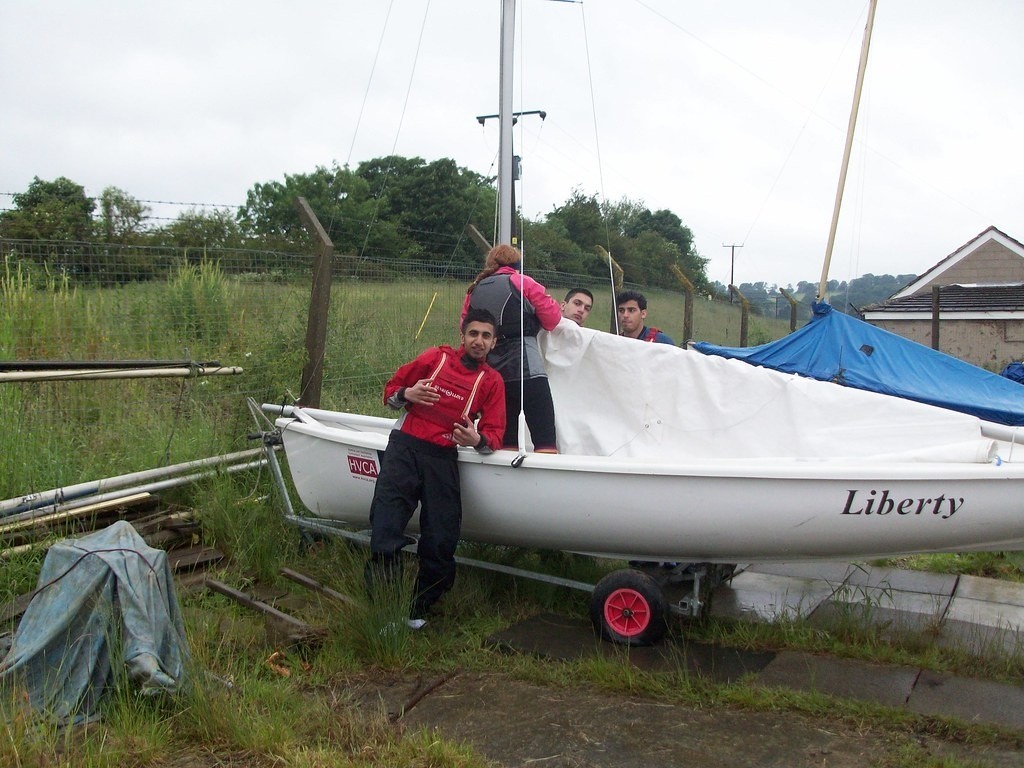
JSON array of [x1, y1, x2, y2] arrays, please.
[[474, 433, 486, 450]]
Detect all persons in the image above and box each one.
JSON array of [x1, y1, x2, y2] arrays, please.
[[459, 245, 562, 454], [559, 287, 594, 327], [364, 307, 507, 632], [615, 291, 675, 345]]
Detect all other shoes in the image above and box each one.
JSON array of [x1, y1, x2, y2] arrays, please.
[[407, 619, 428, 629]]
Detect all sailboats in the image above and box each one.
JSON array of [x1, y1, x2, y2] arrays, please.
[[274, 0, 1024, 565]]
